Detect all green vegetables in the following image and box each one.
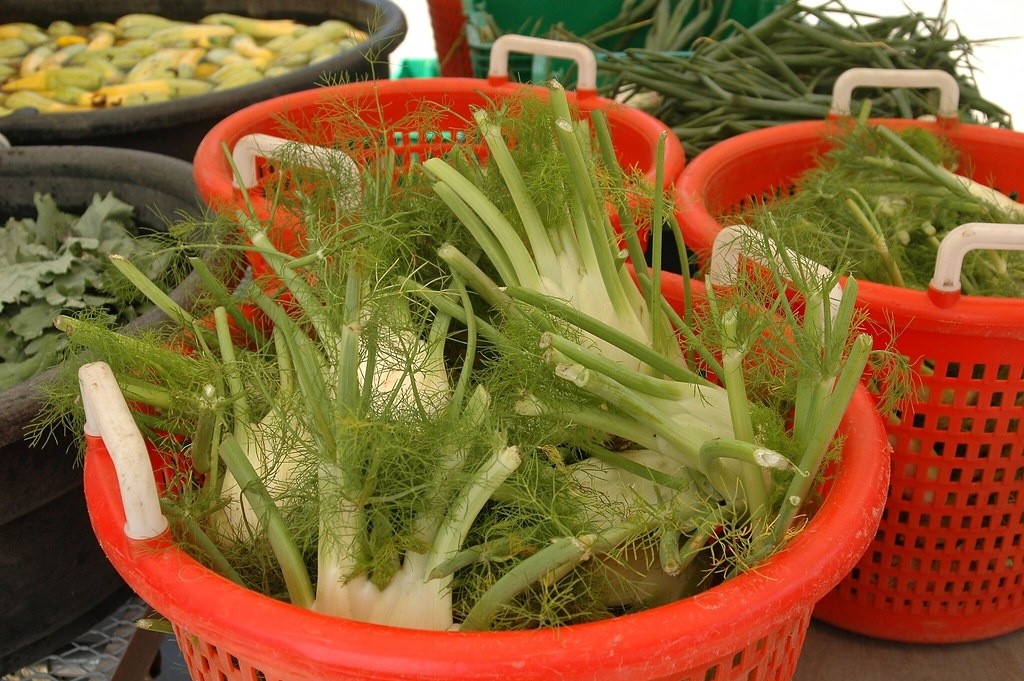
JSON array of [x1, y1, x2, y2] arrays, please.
[[0, 2, 1024, 628]]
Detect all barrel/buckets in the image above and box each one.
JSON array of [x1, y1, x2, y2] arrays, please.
[[0, 0, 408, 164], [0, 145, 220, 681]]
